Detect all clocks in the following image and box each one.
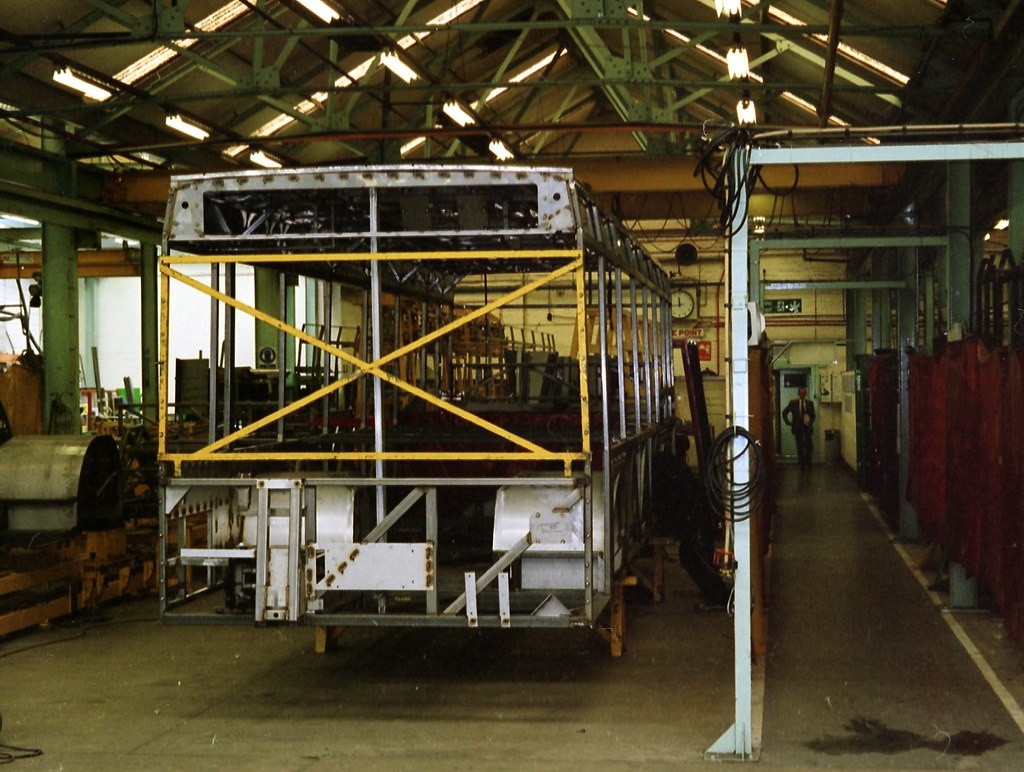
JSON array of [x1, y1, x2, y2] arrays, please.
[[671, 289, 694, 320]]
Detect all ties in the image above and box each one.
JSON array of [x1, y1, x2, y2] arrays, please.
[[801, 400, 805, 418]]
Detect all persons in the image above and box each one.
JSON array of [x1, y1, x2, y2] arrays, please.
[[652, 452, 728, 611], [782, 387, 816, 472]]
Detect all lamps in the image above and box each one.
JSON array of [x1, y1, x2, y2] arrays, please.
[[50, 58, 120, 102], [380, 46, 425, 84], [248, 148, 286, 169], [489, 136, 516, 161], [726, 41, 751, 80], [715, 0, 742, 19], [166, 109, 213, 140], [736, 100, 757, 125], [442, 95, 479, 128], [295, 0, 346, 23]]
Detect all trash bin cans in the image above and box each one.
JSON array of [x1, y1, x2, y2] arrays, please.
[[824, 429, 840, 466]]
